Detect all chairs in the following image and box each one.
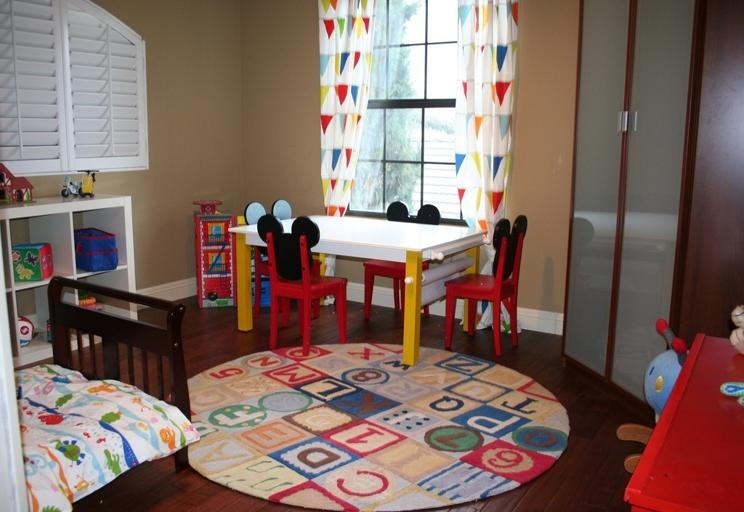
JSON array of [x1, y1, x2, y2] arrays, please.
[[243, 199, 322, 315], [362, 202, 442, 328], [257, 213, 348, 357], [444, 214, 528, 356]]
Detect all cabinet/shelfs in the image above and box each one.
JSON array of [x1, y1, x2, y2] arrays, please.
[[557, 0, 744, 429], [195, 213, 238, 309], [1, 191, 138, 368]]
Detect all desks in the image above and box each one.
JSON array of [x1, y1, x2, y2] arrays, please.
[[229, 215, 489, 366], [626, 330, 744, 512]]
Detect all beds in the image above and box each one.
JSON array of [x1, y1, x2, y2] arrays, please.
[[11, 275, 191, 511]]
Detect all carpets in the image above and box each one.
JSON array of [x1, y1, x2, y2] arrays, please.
[[168, 342, 569, 512]]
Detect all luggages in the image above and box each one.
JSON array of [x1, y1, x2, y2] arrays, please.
[[74, 228, 120, 272]]
